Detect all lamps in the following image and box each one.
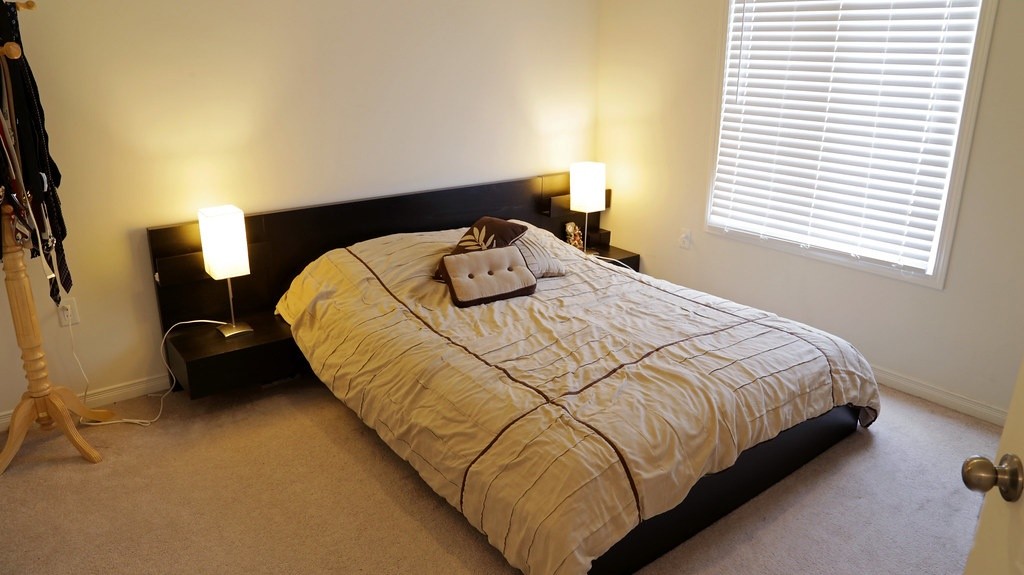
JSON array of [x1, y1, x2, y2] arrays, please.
[[196, 202, 253, 336], [569, 161, 607, 256]]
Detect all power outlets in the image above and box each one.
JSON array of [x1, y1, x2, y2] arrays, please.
[[56, 297, 80, 328]]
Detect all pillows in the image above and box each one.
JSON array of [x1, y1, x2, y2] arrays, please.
[[517, 237, 563, 279], [439, 246, 538, 309], [432, 216, 529, 282]]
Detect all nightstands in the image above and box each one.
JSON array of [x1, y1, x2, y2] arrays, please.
[[162, 319, 298, 402], [586, 239, 640, 271]]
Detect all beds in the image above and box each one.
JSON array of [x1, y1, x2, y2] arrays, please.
[[146, 170, 882, 575]]
